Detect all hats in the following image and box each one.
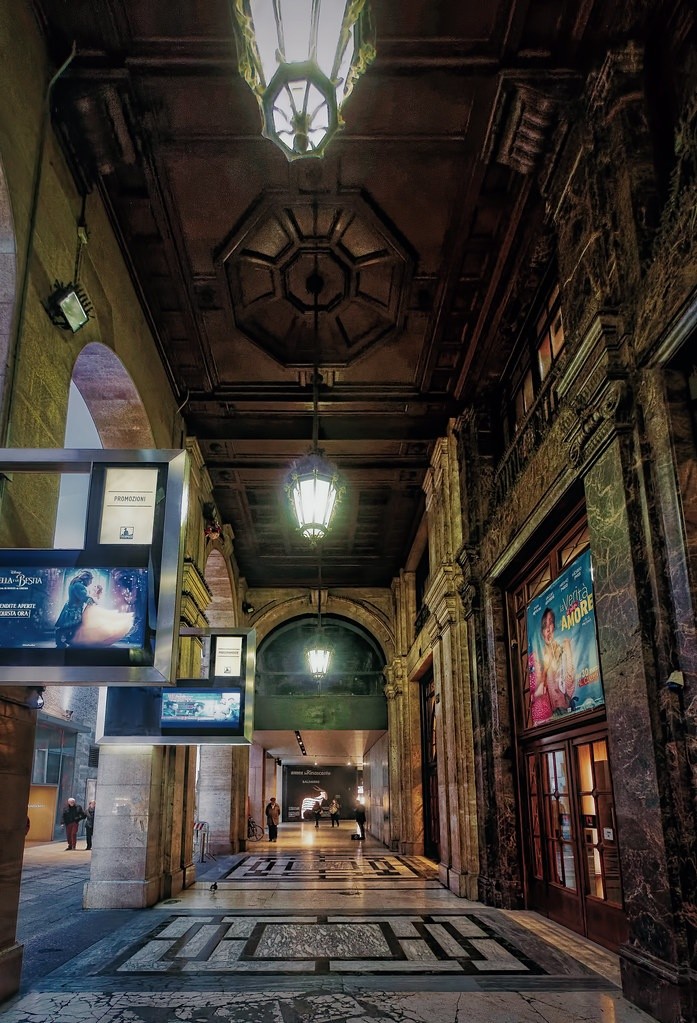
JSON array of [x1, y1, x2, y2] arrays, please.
[[270, 798, 276, 801]]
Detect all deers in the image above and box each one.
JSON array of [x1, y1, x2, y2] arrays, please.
[[301, 785, 328, 819]]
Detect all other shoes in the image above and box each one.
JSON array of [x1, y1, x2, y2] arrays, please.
[[86, 847, 90, 850], [66, 846, 72, 850], [273, 839, 276, 842], [360, 837, 365, 840], [269, 839, 272, 842]]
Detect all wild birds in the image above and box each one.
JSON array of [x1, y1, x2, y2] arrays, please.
[[210, 882, 218, 896]]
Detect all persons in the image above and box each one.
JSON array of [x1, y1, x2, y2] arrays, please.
[[312, 801, 322, 827], [60, 798, 95, 851], [329, 799, 342, 828], [354, 799, 367, 841], [265, 798, 280, 843], [163, 698, 240, 721], [529, 607, 578, 717]]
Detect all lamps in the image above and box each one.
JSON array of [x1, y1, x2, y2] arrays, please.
[[228, 0, 376, 166], [303, 561, 334, 685], [243, 601, 255, 613], [204, 501, 218, 521], [281, 170, 351, 552], [42, 277, 97, 334], [65, 709, 73, 720]]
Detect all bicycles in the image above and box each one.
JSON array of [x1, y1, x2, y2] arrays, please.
[[247, 816, 264, 842]]
